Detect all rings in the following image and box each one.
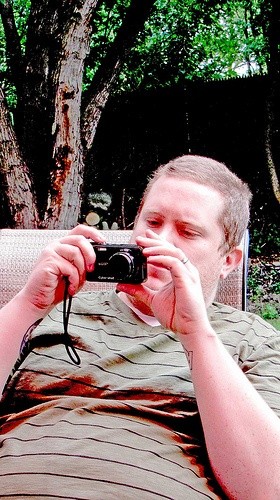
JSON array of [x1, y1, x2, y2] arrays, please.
[[182, 256, 188, 263]]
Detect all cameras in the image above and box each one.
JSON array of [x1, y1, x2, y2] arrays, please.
[[85, 241, 148, 285]]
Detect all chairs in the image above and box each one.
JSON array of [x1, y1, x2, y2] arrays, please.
[[1, 226, 248, 311]]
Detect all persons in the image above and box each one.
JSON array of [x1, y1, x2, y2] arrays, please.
[[77, 210, 124, 231], [0, 153, 280, 499]]
